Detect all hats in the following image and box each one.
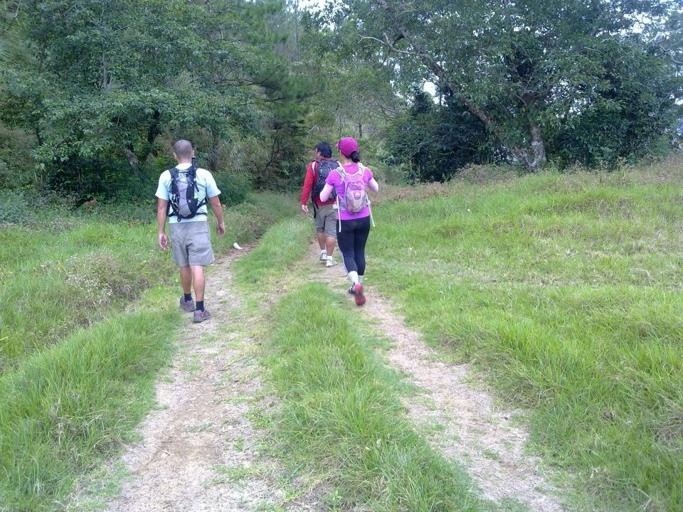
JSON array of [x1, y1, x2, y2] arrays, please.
[[336, 137, 358, 158]]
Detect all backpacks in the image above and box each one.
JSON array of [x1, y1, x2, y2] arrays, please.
[[168, 166, 199, 219], [336, 165, 368, 213], [312, 158, 340, 199]]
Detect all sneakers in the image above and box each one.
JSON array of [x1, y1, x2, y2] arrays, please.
[[180, 296, 194, 311], [348, 282, 366, 305], [320, 253, 337, 266], [194, 310, 209, 323]]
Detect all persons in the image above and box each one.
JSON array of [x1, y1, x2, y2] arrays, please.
[[301, 142, 344, 268], [317, 136, 378, 307], [153, 139, 225, 323]]
